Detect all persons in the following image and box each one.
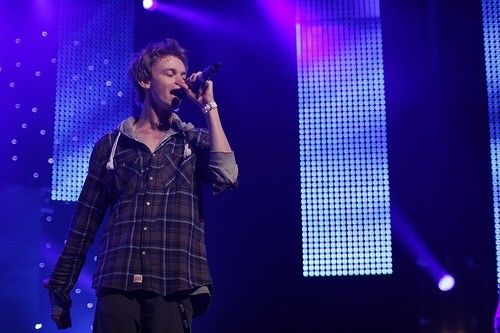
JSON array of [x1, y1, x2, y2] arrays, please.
[[43, 38, 240, 333]]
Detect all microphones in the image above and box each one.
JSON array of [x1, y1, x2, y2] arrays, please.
[[177, 64, 218, 100]]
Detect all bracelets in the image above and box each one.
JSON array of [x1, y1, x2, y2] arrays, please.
[[202, 102, 218, 115]]
